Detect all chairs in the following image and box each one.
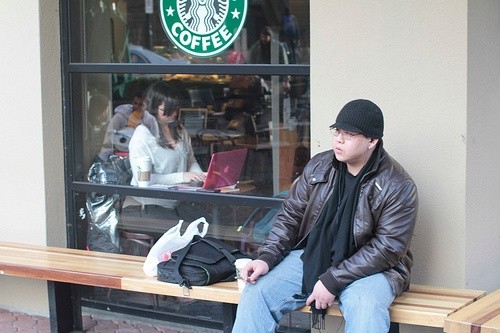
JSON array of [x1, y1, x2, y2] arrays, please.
[[88, 157, 153, 253]]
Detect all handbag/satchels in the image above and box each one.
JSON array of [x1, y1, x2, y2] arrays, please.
[[156, 235, 255, 290], [142, 216, 210, 276]]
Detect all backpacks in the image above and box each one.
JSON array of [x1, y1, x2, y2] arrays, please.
[[237, 190, 290, 245]]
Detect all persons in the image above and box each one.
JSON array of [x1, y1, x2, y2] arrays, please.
[[282, 8, 301, 65], [230, 98, 420, 333], [122, 82, 208, 240], [97, 89, 160, 162], [246, 25, 290, 94]]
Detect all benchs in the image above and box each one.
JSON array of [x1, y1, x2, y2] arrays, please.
[[0, 241, 500, 333], [117, 216, 250, 241]]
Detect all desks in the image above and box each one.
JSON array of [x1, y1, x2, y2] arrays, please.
[[226, 138, 290, 185], [212, 183, 259, 242]]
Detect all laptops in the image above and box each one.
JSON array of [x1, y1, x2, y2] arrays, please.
[[175, 148, 248, 190]]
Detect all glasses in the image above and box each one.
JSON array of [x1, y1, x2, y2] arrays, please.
[[329, 128, 362, 137]]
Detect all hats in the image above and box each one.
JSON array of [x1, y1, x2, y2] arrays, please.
[[329, 99, 384, 139]]
